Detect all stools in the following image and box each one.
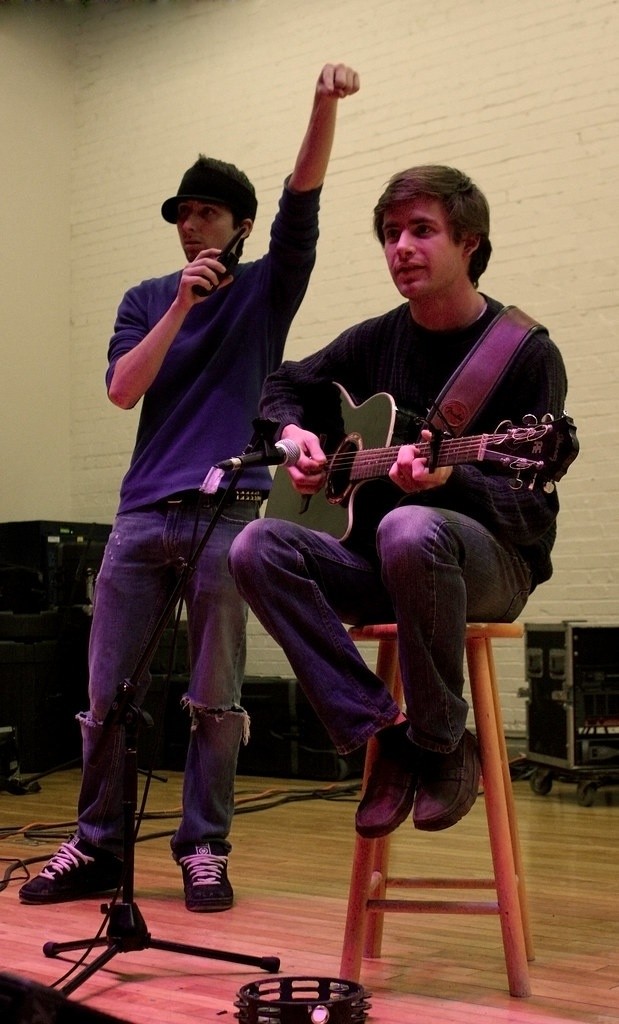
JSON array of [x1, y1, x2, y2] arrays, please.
[[340, 621, 536, 1000]]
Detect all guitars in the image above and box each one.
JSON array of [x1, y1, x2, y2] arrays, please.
[[260, 380, 581, 551]]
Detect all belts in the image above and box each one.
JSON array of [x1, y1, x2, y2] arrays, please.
[[162, 482, 262, 504]]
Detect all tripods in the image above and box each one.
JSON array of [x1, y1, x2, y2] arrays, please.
[[43, 417, 281, 997]]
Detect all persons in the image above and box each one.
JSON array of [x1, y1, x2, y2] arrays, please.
[[17, 62, 360, 913], [228, 164, 579, 839]]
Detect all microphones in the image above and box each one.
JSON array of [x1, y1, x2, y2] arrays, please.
[[215, 440, 301, 473]]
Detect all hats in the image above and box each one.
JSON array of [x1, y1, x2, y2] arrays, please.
[[162, 164, 258, 225]]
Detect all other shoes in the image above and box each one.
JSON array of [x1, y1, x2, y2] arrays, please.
[[354, 713, 414, 839], [414, 726, 481, 831]]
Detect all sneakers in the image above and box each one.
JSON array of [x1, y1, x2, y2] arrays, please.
[[19, 831, 124, 904], [171, 841, 233, 911]]
